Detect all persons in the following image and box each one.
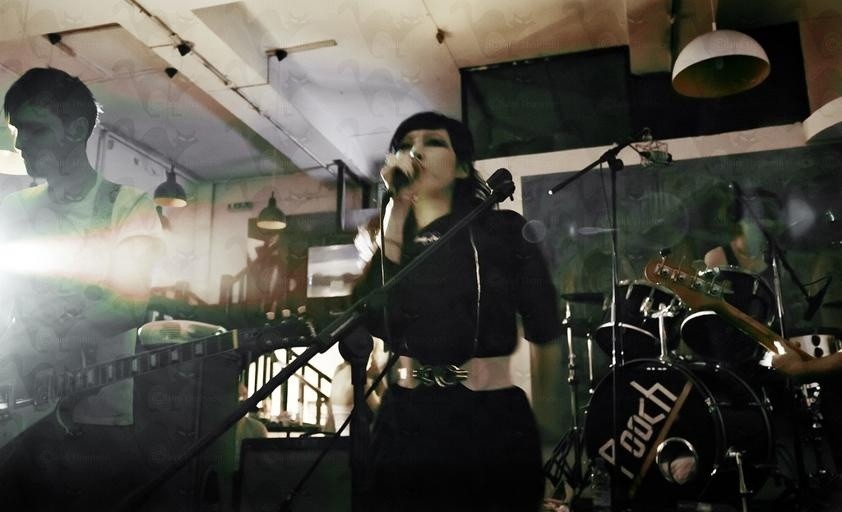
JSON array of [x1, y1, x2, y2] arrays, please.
[[704, 188, 783, 291], [768, 332, 841, 379], [347, 111, 565, 511], [0, 64, 166, 511]]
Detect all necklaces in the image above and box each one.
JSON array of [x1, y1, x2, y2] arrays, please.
[[738, 247, 758, 260]]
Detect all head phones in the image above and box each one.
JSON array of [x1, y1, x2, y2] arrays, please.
[[726, 198, 742, 223]]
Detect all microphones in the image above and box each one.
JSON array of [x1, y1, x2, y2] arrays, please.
[[804, 279, 835, 320], [641, 149, 674, 169], [382, 171, 409, 205]]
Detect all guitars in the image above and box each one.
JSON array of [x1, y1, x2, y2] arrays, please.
[[1, 304, 319, 464]]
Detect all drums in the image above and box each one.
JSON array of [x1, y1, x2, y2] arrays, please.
[[582, 353, 777, 512], [595, 276, 687, 359], [682, 266, 782, 362]]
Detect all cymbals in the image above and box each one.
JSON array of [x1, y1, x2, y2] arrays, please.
[[560, 291, 602, 305]]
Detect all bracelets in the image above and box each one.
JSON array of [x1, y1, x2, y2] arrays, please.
[[378, 227, 404, 249]]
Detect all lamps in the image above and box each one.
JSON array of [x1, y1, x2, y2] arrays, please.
[[672, 0, 770, 97], [255, 48, 288, 229], [152, 42, 190, 208]]
